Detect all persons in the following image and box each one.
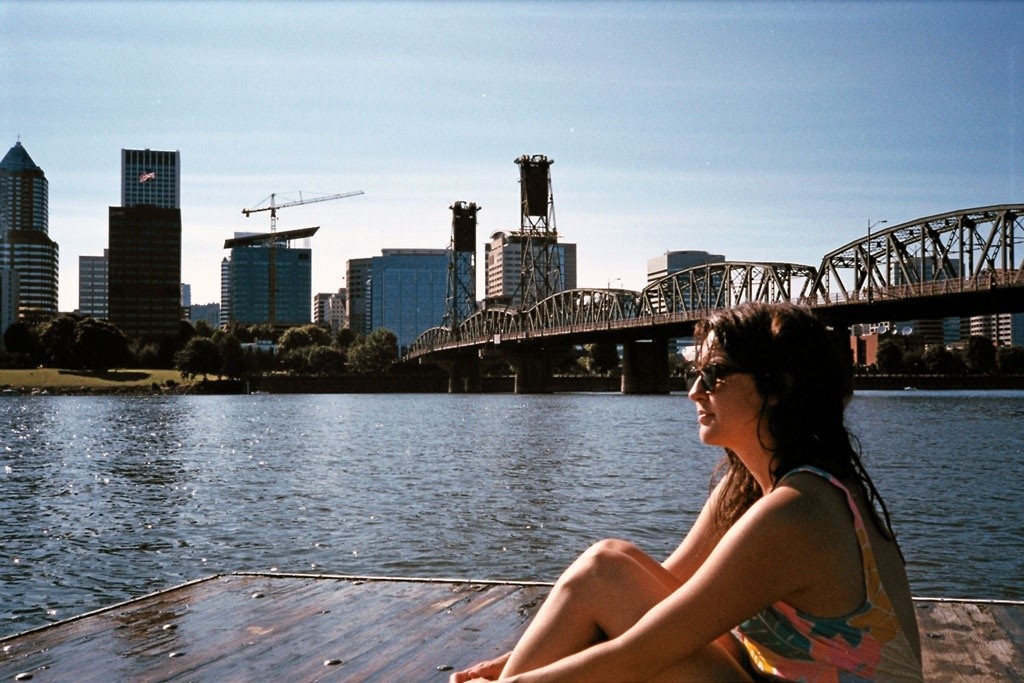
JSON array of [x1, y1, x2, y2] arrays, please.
[[449, 301, 924, 683]]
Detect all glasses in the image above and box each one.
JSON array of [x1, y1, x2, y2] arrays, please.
[[697, 363, 746, 394]]
